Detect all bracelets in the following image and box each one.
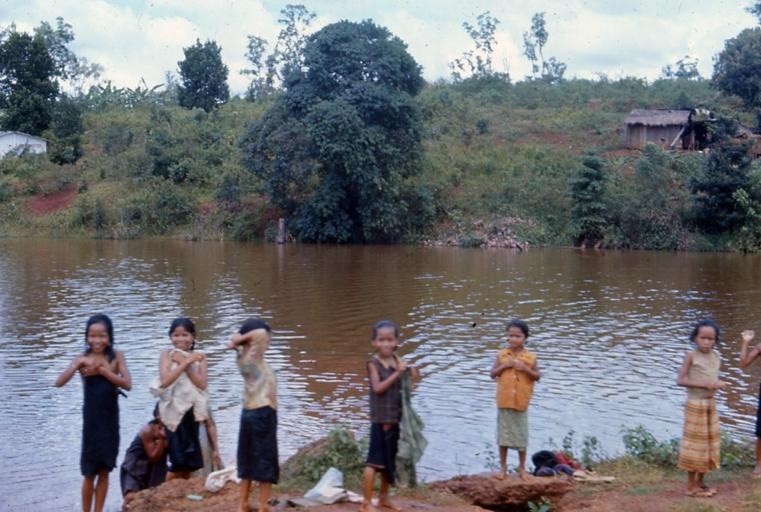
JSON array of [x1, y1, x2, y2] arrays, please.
[[70, 362, 77, 370]]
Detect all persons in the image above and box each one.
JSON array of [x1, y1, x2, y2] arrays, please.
[[190, 405, 223, 478], [675, 317, 729, 497], [223, 316, 282, 511], [55, 314, 134, 512], [488, 318, 543, 482], [148, 316, 212, 480], [118, 399, 172, 511], [360, 321, 424, 512], [738, 328, 761, 479]]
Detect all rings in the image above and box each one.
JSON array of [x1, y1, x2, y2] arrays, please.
[[85, 368, 88, 372]]
